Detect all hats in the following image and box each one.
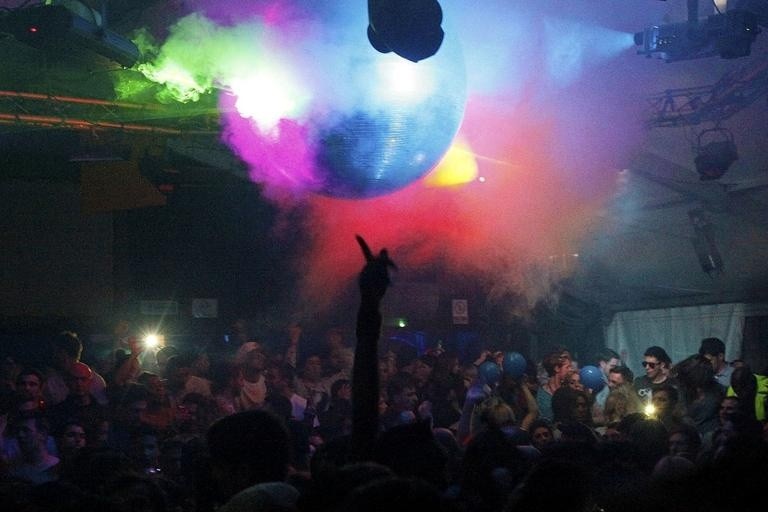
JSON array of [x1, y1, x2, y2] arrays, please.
[[237, 341, 261, 359]]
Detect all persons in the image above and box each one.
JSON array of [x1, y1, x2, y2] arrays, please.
[[1, 233, 767, 512]]
